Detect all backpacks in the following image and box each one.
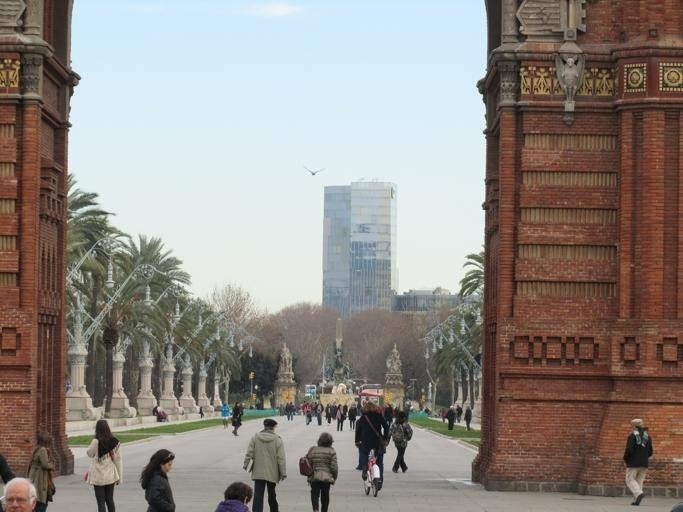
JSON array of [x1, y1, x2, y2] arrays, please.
[[392, 423, 404, 443]]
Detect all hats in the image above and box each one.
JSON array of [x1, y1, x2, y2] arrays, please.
[[264, 419, 278, 427], [631, 418, 643, 428]]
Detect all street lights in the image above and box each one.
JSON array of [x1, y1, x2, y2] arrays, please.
[[66, 237, 254, 424], [416, 293, 482, 427], [409, 377, 417, 418]]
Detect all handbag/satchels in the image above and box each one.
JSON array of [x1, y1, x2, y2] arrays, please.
[[378, 435, 388, 449], [299, 458, 313, 476]]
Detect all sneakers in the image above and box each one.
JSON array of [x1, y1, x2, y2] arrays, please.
[[402, 466, 408, 472], [630, 492, 645, 506], [232, 431, 239, 436], [376, 481, 382, 490], [392, 467, 397, 473], [362, 474, 367, 480]]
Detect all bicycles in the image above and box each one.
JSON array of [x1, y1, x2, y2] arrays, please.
[[357, 443, 381, 496]]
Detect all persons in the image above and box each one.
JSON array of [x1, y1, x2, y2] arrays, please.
[[425, 406, 429, 413], [463, 405, 473, 431], [152, 406, 159, 416], [306, 432, 337, 512], [198, 404, 204, 419], [455, 403, 463, 422], [211, 480, 253, 512], [231, 401, 243, 437], [621, 414, 654, 505], [446, 407, 457, 431], [242, 418, 288, 511], [220, 400, 230, 431], [284, 397, 414, 491], [84, 418, 122, 511], [158, 408, 170, 423], [27, 430, 56, 512], [439, 405, 446, 422], [0, 453, 17, 512], [0, 476, 44, 512], [138, 448, 176, 512]]
